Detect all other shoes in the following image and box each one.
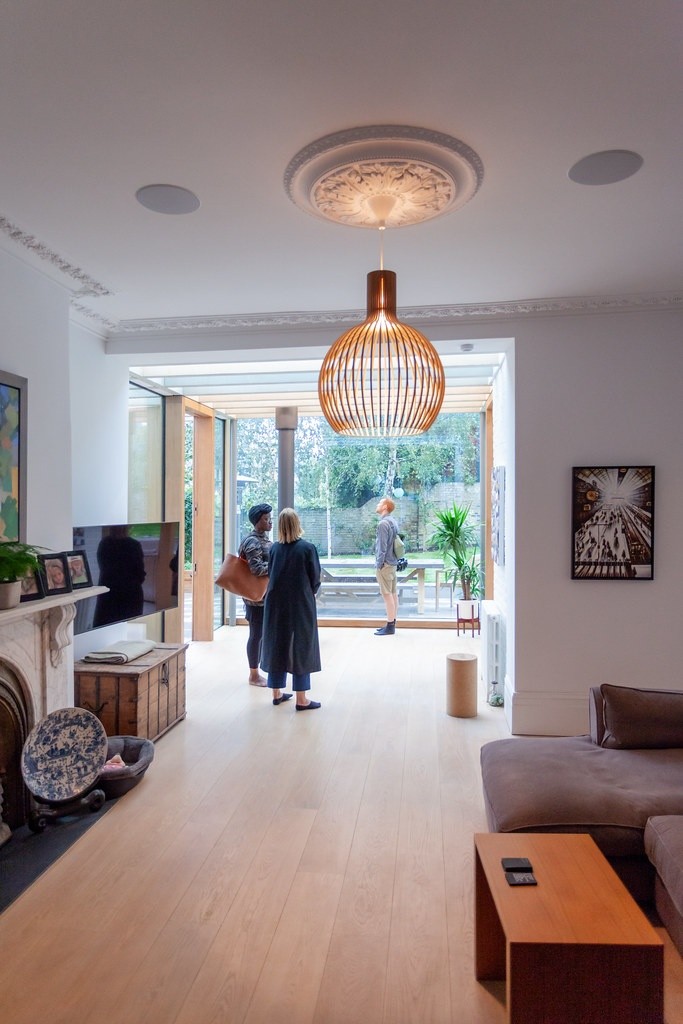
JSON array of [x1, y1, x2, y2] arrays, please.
[[295, 700, 321, 711], [373, 619, 396, 635], [273, 693, 293, 705]]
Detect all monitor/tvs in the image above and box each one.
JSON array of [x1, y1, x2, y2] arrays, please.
[[72, 522, 180, 637]]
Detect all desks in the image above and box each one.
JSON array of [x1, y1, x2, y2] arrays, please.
[[317, 558, 444, 614]]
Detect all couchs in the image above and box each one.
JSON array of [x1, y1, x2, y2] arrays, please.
[[479, 685, 683, 907], [643, 814, 683, 958]]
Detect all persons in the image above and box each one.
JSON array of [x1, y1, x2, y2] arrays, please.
[[259, 507, 321, 710], [46, 560, 66, 590], [69, 557, 88, 584], [93, 525, 147, 627], [238, 504, 274, 687], [374, 497, 399, 635]]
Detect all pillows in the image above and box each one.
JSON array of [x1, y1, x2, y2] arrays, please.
[[599, 683, 683, 750]]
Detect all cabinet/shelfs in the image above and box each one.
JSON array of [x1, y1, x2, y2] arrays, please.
[[74, 643, 188, 742]]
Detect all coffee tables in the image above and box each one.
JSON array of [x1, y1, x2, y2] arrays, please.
[[474, 833, 665, 1024]]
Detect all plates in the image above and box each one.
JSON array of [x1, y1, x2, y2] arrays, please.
[[20, 707, 107, 801]]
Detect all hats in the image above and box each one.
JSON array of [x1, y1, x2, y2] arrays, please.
[[248, 503, 272, 526]]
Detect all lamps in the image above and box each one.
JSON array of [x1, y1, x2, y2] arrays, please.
[[318, 195, 445, 438]]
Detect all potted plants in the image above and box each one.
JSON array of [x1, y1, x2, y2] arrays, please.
[[0, 541, 54, 610], [424, 497, 485, 619]]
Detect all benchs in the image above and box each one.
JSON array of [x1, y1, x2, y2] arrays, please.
[[315, 574, 462, 608]]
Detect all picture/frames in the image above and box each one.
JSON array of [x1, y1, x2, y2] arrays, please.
[[571, 464, 654, 582], [0, 369, 29, 547], [36, 551, 74, 595], [60, 550, 94, 589], [16, 563, 45, 602]]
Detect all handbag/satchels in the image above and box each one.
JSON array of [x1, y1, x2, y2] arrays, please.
[[394, 534, 406, 560], [214, 550, 270, 603]]
